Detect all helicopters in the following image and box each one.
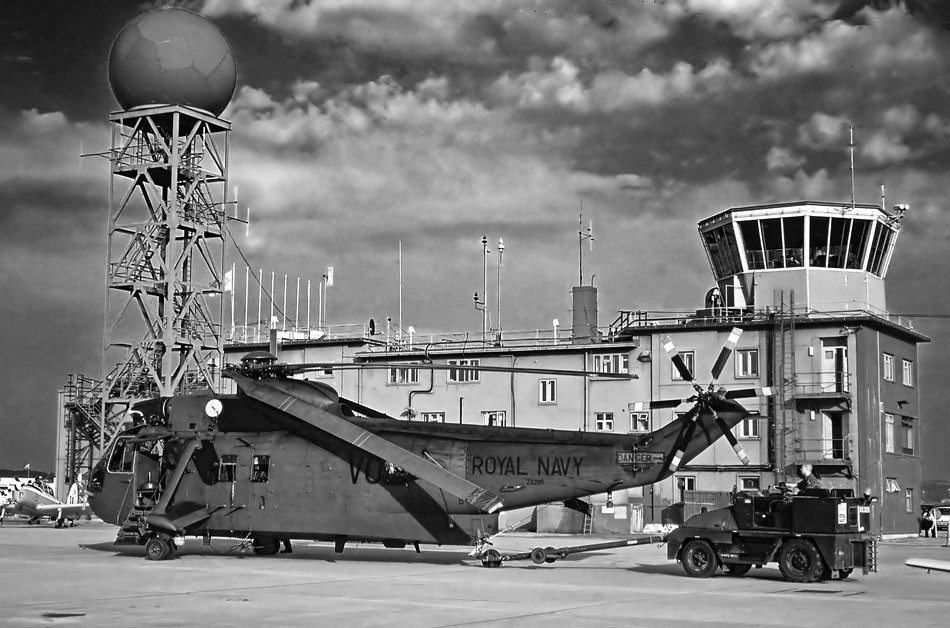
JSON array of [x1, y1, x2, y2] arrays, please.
[[78, 327, 779, 563]]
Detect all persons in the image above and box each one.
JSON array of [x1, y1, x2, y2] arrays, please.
[[785, 464, 821, 495], [280, 537, 293, 553]]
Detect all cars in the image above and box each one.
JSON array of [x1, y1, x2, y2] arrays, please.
[[936, 506, 950, 531]]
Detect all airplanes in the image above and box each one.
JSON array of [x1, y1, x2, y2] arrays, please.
[[0, 482, 93, 528]]
